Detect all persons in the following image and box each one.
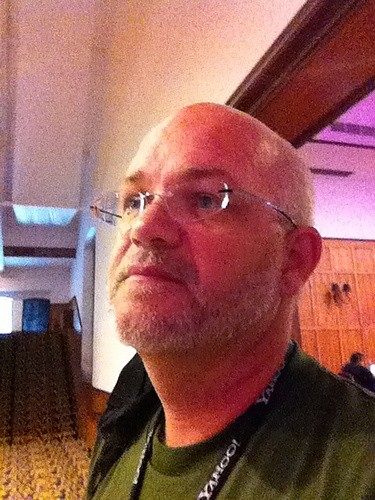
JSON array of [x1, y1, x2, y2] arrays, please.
[[80, 103, 375, 500], [340, 350, 375, 393]]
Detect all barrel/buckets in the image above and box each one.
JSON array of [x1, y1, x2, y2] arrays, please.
[[21, 298, 51, 334]]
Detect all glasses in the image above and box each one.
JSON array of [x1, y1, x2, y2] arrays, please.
[[87, 180, 297, 229]]
